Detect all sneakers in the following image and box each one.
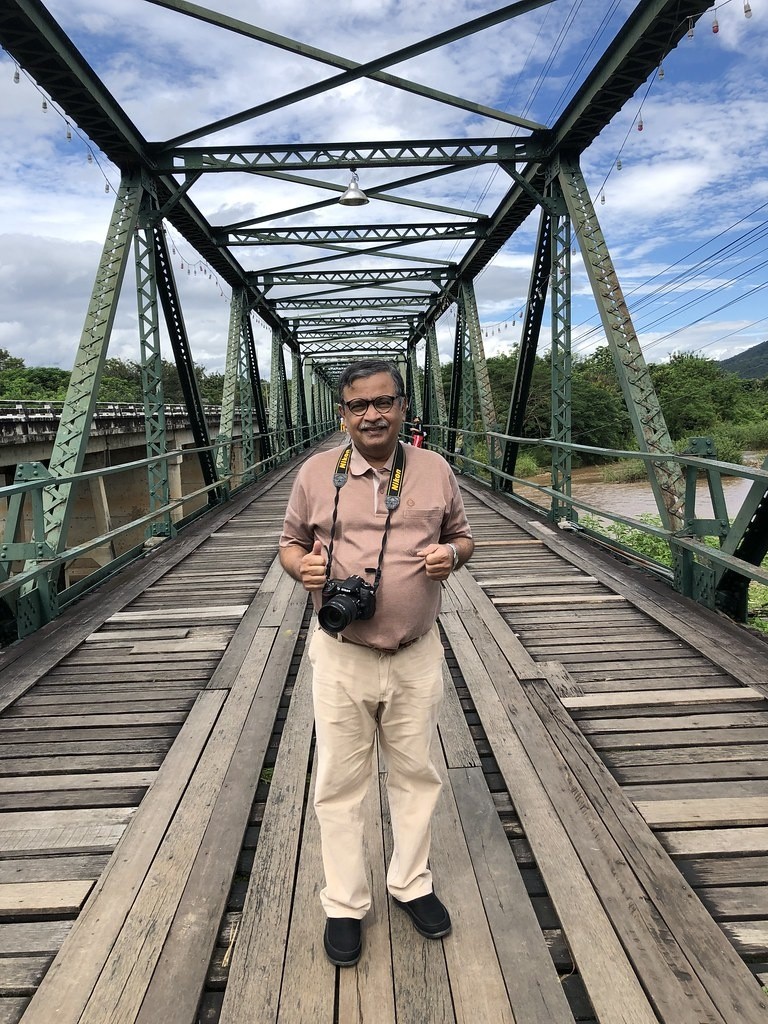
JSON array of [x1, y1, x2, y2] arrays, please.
[[392, 890, 452, 940], [324, 918, 364, 967]]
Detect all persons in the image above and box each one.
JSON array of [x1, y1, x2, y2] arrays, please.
[[339, 416, 346, 433], [409, 416, 425, 448], [278, 359, 474, 967]]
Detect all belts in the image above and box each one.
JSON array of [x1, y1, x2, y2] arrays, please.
[[320, 627, 418, 655]]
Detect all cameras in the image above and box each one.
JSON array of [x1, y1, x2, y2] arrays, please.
[[317, 575, 376, 634]]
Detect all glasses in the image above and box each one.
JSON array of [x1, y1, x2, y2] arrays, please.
[[342, 393, 403, 416]]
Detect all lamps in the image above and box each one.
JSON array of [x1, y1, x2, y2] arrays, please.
[[336, 167, 370, 206]]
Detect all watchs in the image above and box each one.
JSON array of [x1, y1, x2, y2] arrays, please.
[[447, 542, 460, 571]]
